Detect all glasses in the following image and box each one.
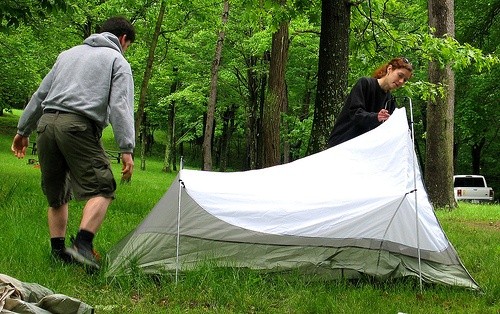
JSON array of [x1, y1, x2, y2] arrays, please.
[[396, 57, 413, 67]]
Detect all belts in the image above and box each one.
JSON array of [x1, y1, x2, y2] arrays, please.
[[43, 109, 69, 114]]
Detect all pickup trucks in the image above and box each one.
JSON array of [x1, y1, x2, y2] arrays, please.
[[453, 174, 495, 204]]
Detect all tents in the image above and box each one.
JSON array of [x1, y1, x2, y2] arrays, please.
[[103, 107, 480, 290]]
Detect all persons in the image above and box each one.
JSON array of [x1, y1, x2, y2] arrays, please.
[[11, 16, 135, 272], [328, 58, 412, 150]]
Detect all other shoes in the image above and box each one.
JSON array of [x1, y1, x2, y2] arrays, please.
[[49, 250, 72, 266], [65, 240, 100, 270]]
